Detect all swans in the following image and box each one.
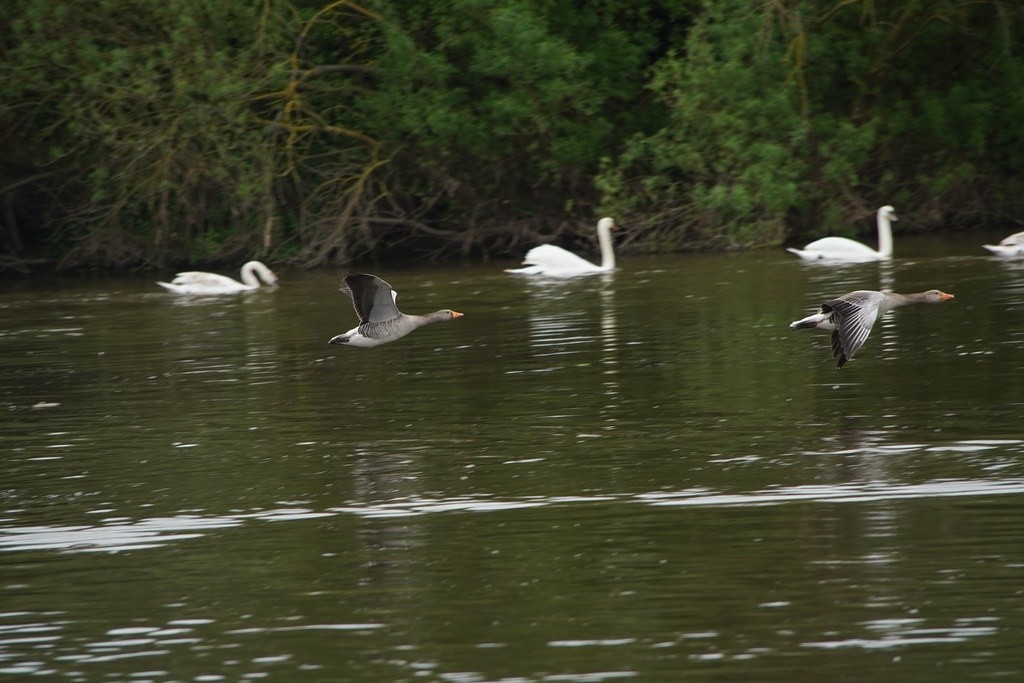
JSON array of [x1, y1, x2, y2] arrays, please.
[[154, 259, 281, 297], [501, 217, 621, 279], [786, 205, 899, 262], [981, 232, 1024, 257]]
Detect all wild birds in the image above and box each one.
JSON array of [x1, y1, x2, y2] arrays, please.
[[329, 270, 464, 348], [793, 289, 954, 370]]
